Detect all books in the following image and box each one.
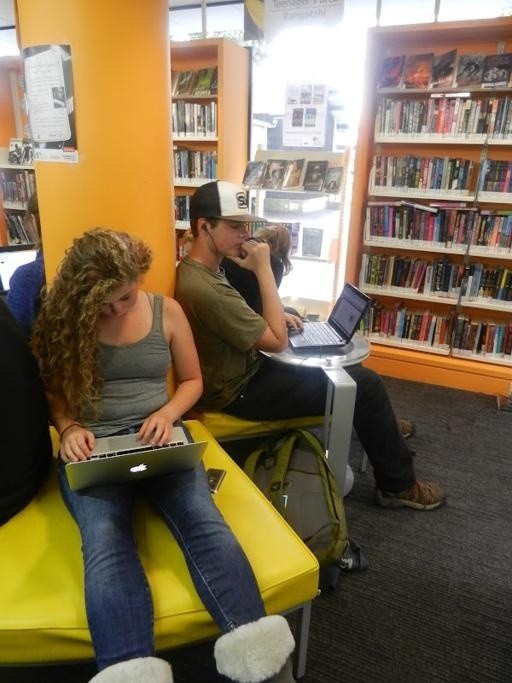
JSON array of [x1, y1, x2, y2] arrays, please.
[[0, 137, 39, 243], [243, 162, 343, 258], [170, 69, 219, 259]]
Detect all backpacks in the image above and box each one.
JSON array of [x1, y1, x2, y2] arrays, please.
[[243, 428, 369, 602]]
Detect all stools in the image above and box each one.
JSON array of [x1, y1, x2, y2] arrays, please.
[[195, 402, 351, 566], [0, 418, 325, 682]]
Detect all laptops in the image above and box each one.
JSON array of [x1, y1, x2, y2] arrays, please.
[[287, 283, 371, 350], [66, 427, 209, 493]]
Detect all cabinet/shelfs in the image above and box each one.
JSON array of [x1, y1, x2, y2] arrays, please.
[[342, 10, 512, 399], [0, 163, 37, 213], [168, 35, 251, 260]]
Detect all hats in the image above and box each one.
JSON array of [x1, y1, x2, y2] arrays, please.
[[189, 180, 268, 225]]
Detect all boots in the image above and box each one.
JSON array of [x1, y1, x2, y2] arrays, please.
[[88, 656, 174, 683], [214, 614, 297, 683]]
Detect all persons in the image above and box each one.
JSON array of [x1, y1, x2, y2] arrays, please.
[[6, 193, 46, 337], [175, 180, 445, 510], [0, 295, 51, 524], [226, 225, 292, 313], [31, 228, 300, 683]]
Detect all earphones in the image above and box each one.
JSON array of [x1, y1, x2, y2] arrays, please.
[[201, 224, 207, 230]]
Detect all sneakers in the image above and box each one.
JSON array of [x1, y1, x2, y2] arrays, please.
[[396, 417, 414, 440], [373, 478, 445, 511]]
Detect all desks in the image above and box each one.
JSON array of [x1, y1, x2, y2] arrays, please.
[[255, 331, 373, 495]]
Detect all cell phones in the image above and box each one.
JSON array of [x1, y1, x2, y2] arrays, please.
[[206, 468, 226, 493]]
